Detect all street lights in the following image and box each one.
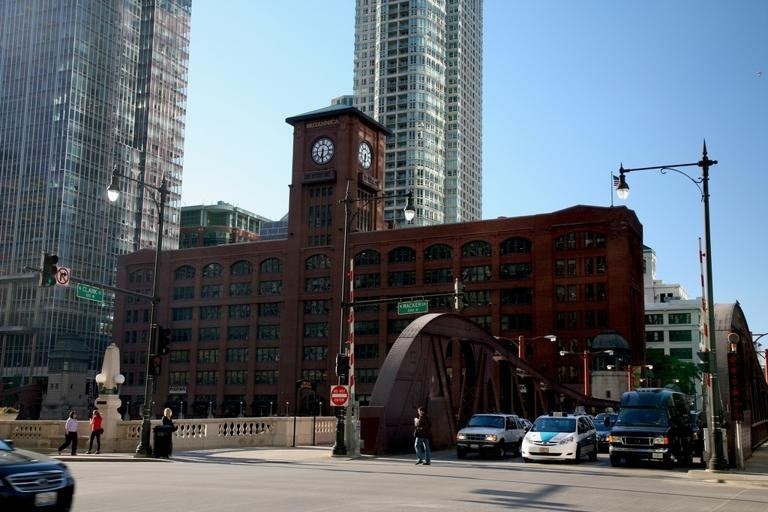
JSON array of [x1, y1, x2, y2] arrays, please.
[[615, 137, 732, 474], [607, 364, 654, 392], [559, 349, 615, 398], [122, 398, 323, 420], [106, 165, 172, 459], [331, 180, 416, 457], [492, 335, 557, 361], [640, 378, 680, 388]]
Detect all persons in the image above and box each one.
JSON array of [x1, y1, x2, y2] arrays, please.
[[58, 411, 79, 456], [162, 408, 178, 456], [86, 410, 103, 454], [414, 407, 431, 465]]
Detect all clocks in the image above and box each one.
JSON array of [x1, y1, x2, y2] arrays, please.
[[311, 136, 335, 165], [358, 142, 373, 169]]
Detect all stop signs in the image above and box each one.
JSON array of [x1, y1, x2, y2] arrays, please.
[[330, 385, 349, 407]]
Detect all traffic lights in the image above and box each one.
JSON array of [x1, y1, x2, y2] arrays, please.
[[153, 325, 171, 358], [696, 350, 715, 374], [38, 252, 58, 287], [337, 354, 351, 378]]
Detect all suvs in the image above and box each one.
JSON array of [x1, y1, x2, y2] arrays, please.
[[456, 413, 524, 460]]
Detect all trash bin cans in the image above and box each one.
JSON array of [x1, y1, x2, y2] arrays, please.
[[152, 425, 173, 458]]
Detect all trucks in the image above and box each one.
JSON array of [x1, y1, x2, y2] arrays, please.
[[609, 390, 691, 469]]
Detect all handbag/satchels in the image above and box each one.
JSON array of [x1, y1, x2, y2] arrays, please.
[[414, 427, 423, 437]]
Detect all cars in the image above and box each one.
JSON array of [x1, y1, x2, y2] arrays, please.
[[0, 439, 75, 512], [519, 409, 706, 457], [521, 412, 598, 464]]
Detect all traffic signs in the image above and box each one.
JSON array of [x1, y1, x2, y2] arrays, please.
[[398, 300, 429, 315], [77, 284, 104, 303]]
